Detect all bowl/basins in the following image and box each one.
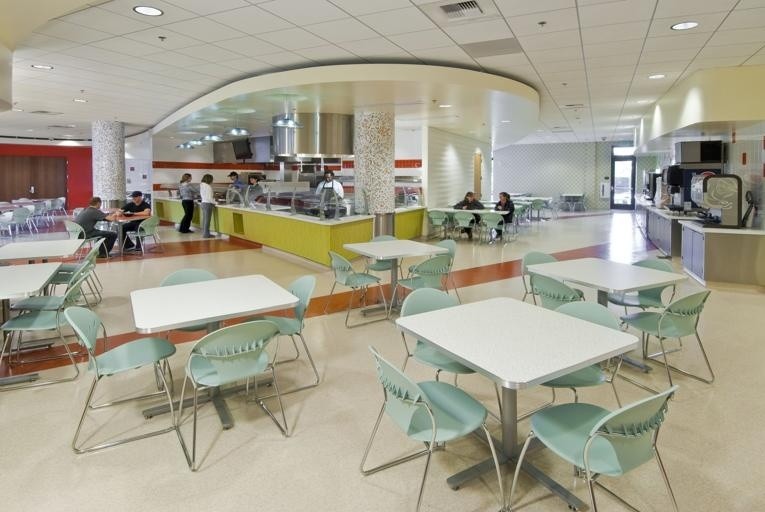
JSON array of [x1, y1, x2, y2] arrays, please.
[[123, 214, 134, 217]]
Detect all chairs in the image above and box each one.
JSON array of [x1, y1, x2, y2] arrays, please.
[[0, 195, 166, 393], [426, 192, 590, 245]]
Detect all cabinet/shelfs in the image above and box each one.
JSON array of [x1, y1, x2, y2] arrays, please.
[[681, 225, 703, 279]]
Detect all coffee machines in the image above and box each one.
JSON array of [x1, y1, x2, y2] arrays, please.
[[664, 168, 723, 212]]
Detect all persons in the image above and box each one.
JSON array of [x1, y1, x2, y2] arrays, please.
[[178, 173, 200, 233], [315, 170, 345, 203], [116, 190, 152, 252], [215, 171, 244, 201], [246, 175, 264, 202], [73, 197, 118, 257], [199, 174, 218, 238], [453, 192, 484, 241], [494, 191, 515, 238]]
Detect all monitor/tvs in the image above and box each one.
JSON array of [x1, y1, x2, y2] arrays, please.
[[232, 138, 253, 160]]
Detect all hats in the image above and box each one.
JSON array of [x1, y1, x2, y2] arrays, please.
[[227, 171, 237, 176], [129, 191, 143, 197]]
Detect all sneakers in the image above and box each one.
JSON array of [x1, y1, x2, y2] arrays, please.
[[181, 228, 215, 238], [462, 229, 472, 238], [496, 232, 502, 238], [126, 245, 141, 252]]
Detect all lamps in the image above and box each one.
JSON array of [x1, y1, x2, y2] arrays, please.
[[271, 98, 304, 129], [229, 115, 252, 137]]
